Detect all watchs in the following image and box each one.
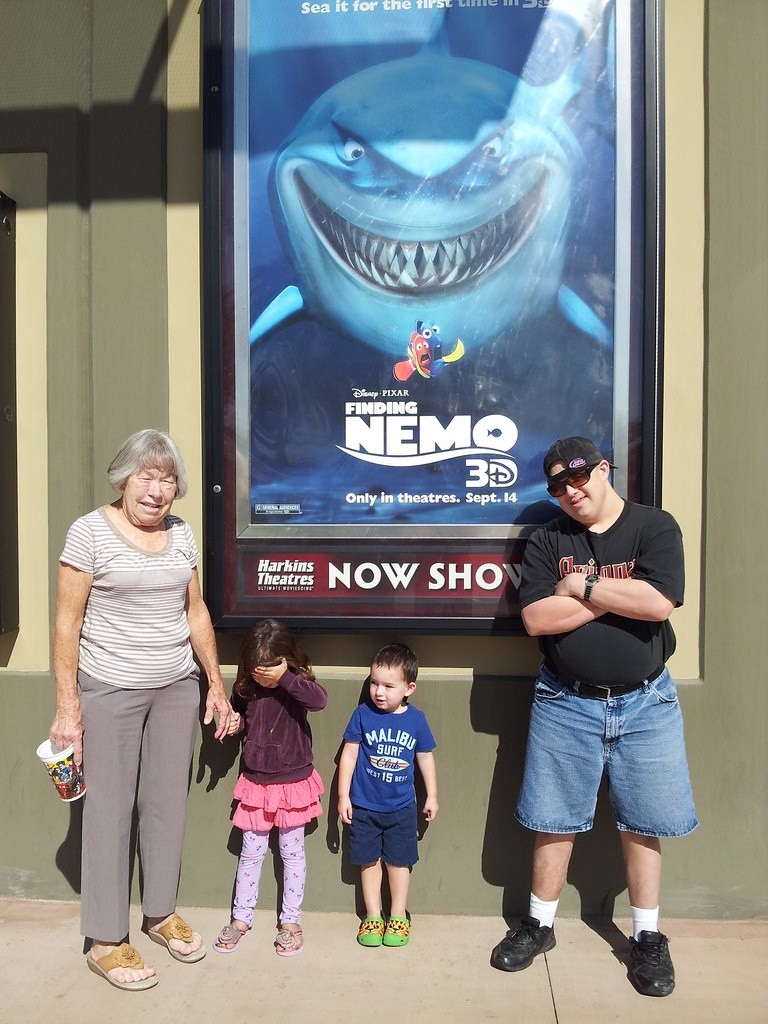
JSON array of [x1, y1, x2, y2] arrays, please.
[[584, 574, 601, 602]]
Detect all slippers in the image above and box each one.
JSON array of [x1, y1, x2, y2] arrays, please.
[[148, 915, 207, 963], [213, 925, 252, 953], [276, 928, 304, 956], [87, 943, 158, 991]]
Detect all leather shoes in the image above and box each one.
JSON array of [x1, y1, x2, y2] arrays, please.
[[630, 929, 674, 997], [491, 915, 556, 972]]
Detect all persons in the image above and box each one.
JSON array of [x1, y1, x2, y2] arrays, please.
[[489, 437, 682, 997], [49, 430, 235, 991], [211, 619, 329, 956], [337, 645, 440, 947]]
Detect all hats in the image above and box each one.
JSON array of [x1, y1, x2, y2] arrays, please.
[[543, 436, 619, 486]]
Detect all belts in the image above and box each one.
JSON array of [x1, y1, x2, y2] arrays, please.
[[542, 656, 666, 701]]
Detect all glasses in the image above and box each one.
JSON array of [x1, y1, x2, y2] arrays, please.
[[547, 465, 596, 497]]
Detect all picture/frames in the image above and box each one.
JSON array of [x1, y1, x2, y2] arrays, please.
[[203, 1, 668, 638]]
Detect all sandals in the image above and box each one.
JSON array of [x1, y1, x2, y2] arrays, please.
[[382, 909, 411, 947], [357, 910, 386, 947]]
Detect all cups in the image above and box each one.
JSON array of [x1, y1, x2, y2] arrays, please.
[[36, 738, 87, 802]]
